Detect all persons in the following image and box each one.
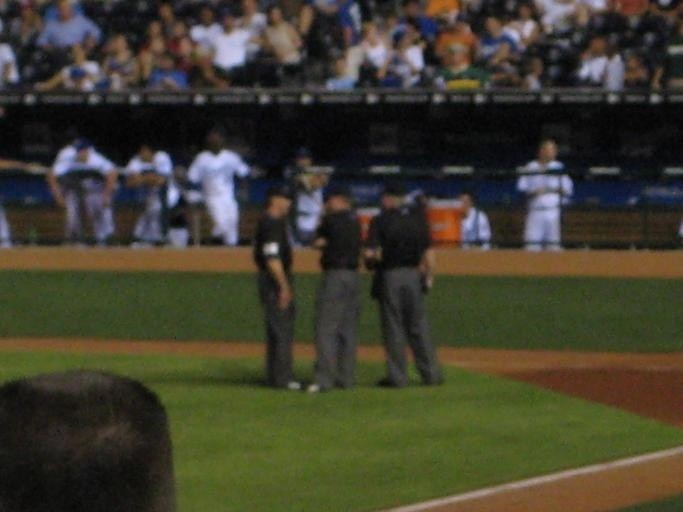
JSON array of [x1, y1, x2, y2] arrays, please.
[[251, 182, 298, 388], [0, 368, 179, 510], [301, 185, 358, 391], [360, 183, 441, 388]]
